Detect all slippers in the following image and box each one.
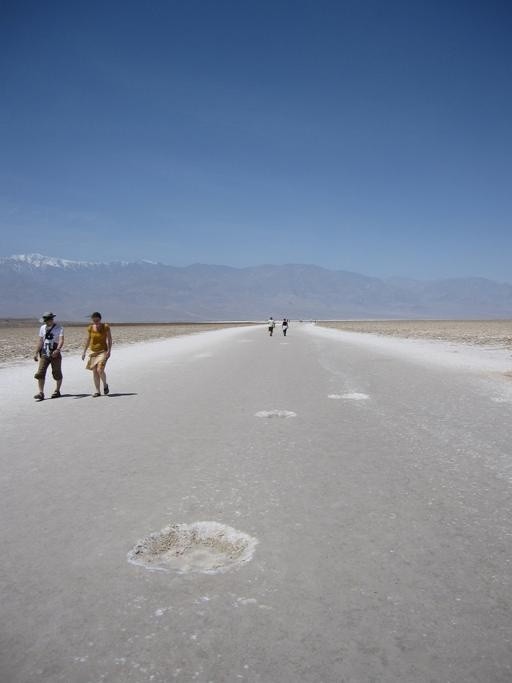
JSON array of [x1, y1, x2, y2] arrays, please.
[[103, 384, 109, 393], [92, 392, 101, 396]]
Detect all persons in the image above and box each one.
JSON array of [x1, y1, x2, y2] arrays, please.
[[81, 312, 112, 396], [268, 316, 274, 336], [34, 311, 64, 399], [282, 318, 288, 335]]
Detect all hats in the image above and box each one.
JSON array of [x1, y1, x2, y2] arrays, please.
[[42, 312, 56, 321]]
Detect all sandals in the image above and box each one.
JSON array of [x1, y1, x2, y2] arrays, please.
[[51, 390, 60, 398], [34, 392, 44, 400]]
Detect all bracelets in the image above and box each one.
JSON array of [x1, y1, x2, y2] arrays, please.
[[56, 348, 61, 352]]
